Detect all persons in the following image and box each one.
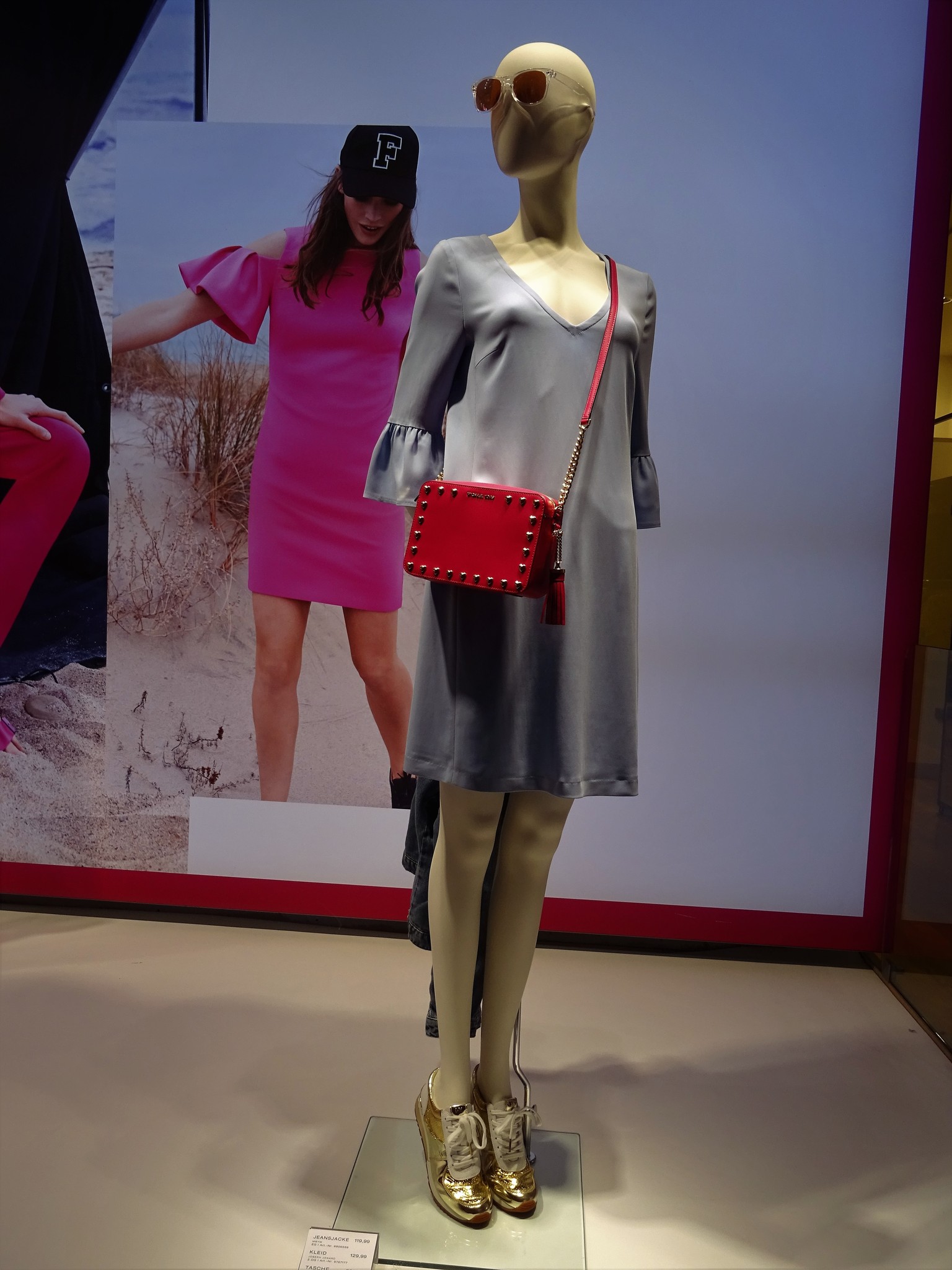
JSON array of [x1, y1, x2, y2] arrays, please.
[[362, 43, 660, 1226], [0, 386, 90, 755], [110, 124, 430, 810]]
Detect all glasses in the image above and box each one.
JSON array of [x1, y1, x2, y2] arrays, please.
[[353, 197, 398, 207], [472, 69, 596, 150]]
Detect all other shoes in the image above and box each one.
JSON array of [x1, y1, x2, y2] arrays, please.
[[389, 770, 413, 809]]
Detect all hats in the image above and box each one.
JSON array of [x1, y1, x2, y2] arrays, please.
[[340, 125, 419, 209]]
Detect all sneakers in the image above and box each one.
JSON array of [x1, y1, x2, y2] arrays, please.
[[414, 1068, 494, 1228], [476, 1066, 536, 1219]]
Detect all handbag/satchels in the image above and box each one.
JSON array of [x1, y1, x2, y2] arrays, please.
[[403, 481, 564, 599]]
